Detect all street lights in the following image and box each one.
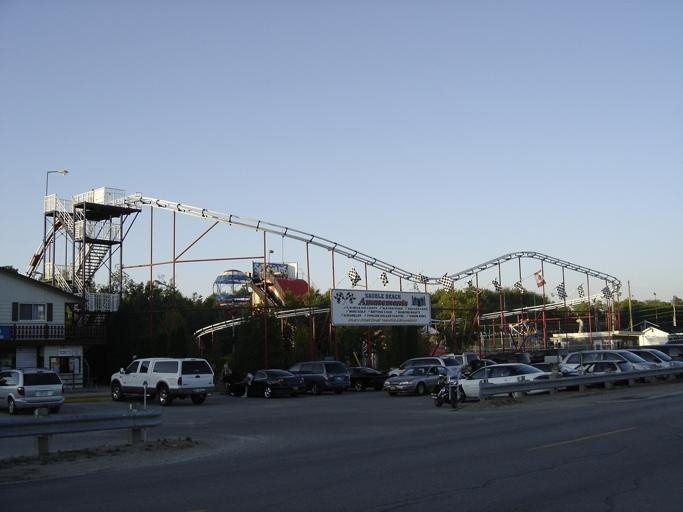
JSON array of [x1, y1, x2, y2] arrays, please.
[[268, 248, 275, 267], [652, 291, 657, 324], [42, 168, 70, 281]]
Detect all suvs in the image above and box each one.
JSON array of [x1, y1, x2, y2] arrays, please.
[[110, 356, 216, 405], [387, 357, 447, 378], [0, 366, 65, 415]]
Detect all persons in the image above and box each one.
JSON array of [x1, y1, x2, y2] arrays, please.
[[222, 363, 232, 395], [239, 369, 255, 398]]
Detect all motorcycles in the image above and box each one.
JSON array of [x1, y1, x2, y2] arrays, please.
[[429, 369, 462, 410]]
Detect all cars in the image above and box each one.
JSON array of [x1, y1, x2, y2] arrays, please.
[[440, 347, 683, 401], [347, 366, 388, 393], [228, 369, 306, 399], [384, 365, 449, 397]]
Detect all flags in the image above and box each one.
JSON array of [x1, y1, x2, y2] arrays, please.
[[514, 282, 523, 290], [347, 267, 362, 287], [534, 271, 546, 288], [491, 279, 502, 291], [379, 272, 389, 287], [556, 282, 567, 299], [601, 286, 612, 299], [577, 285, 584, 298], [440, 276, 453, 289]]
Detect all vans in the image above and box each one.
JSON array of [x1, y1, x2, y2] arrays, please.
[[287, 360, 351, 396]]
